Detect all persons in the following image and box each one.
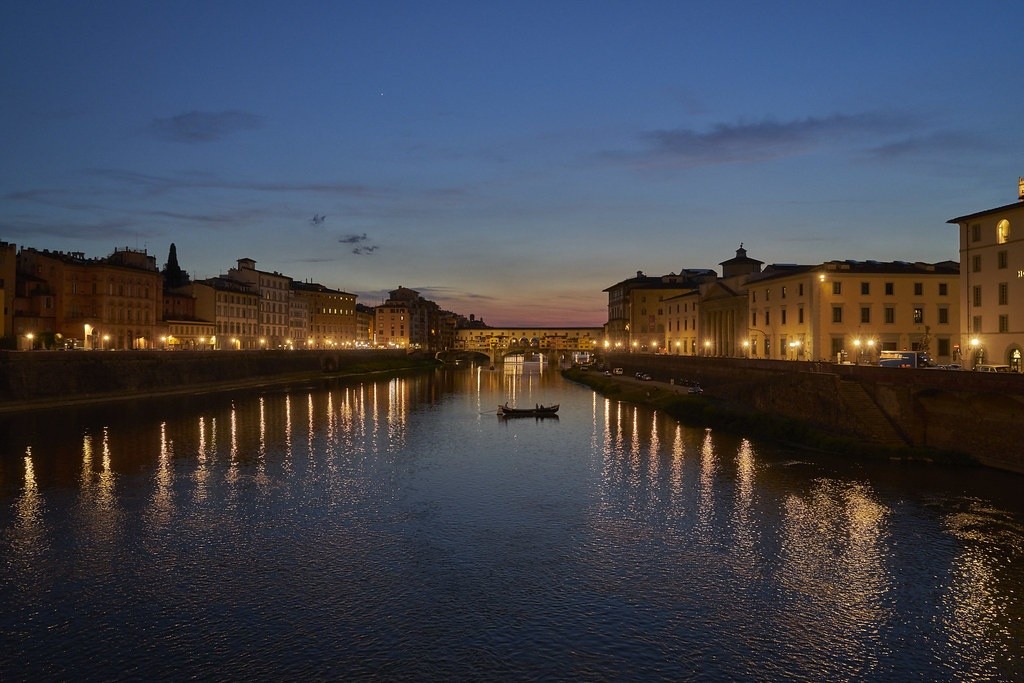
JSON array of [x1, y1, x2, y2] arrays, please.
[[505, 401, 545, 409]]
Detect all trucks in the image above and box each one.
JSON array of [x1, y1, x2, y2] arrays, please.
[[879, 350, 934, 368]]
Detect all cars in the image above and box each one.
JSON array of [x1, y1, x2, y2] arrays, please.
[[579, 363, 610, 372], [635, 372, 652, 381], [675, 376, 699, 387], [602, 370, 612, 377], [687, 387, 703, 394], [934, 364, 962, 369]]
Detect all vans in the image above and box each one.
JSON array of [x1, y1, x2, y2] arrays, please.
[[974, 364, 1013, 372], [613, 368, 623, 376]]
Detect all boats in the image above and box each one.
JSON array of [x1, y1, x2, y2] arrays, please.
[[496, 402, 560, 416], [490, 366, 494, 370]]
